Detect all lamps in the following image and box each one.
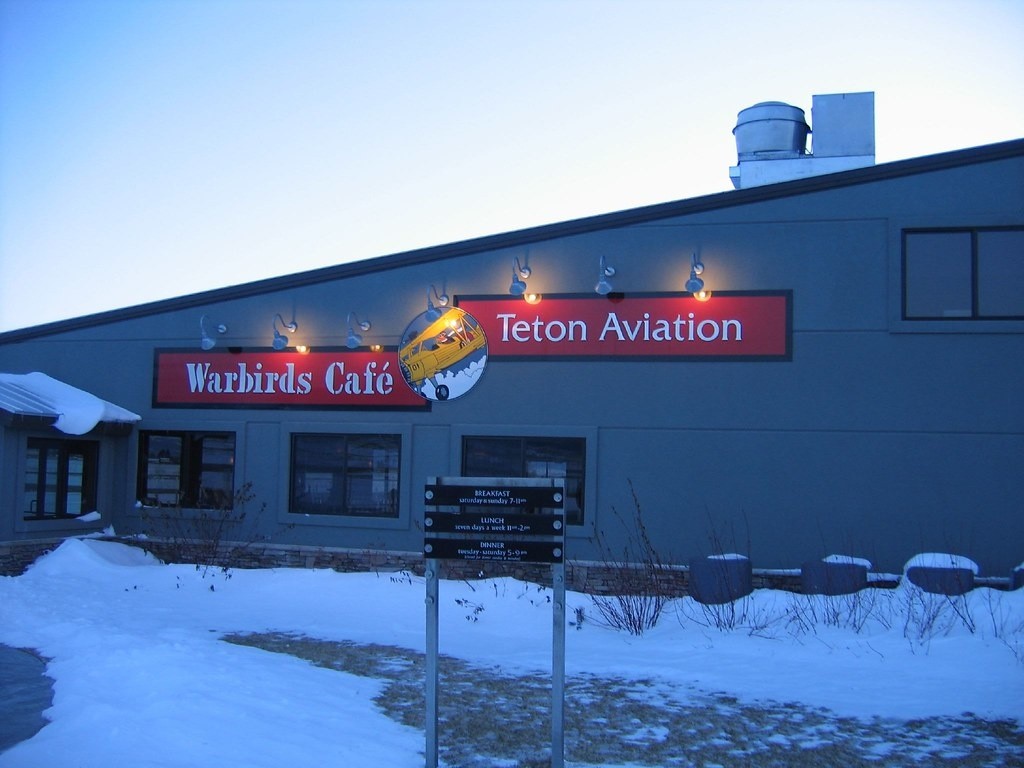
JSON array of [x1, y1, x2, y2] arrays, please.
[[592, 255, 619, 298], [509, 256, 531, 294], [272, 313, 296, 351], [685, 255, 703, 293], [343, 310, 369, 350], [426, 285, 461, 332], [199, 314, 226, 350]]
